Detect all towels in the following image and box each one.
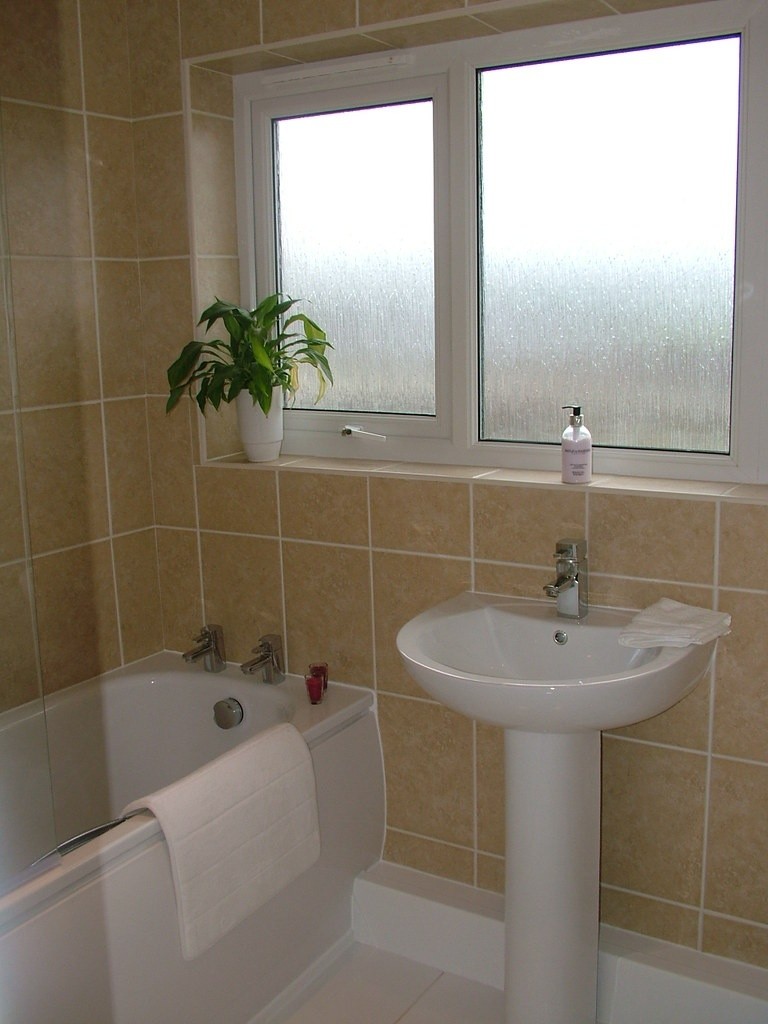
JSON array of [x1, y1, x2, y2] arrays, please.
[[617, 595, 735, 649], [117, 719, 325, 960]]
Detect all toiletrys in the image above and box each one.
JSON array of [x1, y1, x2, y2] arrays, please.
[[560, 403, 593, 483]]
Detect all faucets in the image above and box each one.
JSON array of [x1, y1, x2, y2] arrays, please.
[[182, 623, 227, 673], [543, 538, 587, 617], [240, 633, 287, 685]]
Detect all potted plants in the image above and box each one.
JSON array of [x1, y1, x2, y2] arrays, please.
[[164, 292, 336, 463]]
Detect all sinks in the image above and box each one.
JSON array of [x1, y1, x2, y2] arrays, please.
[[0, 648, 387, 1024], [394, 587, 720, 732]]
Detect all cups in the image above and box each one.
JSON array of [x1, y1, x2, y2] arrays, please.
[[304, 673, 323, 705], [309, 662, 329, 693]]
